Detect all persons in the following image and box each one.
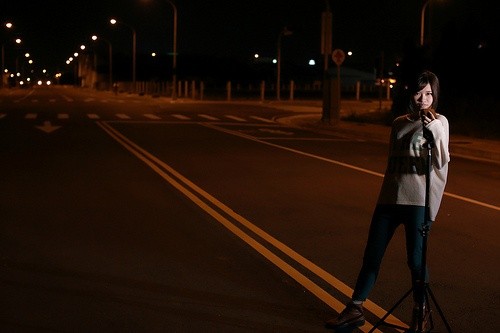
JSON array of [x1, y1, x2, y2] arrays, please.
[[324, 70, 450, 333]]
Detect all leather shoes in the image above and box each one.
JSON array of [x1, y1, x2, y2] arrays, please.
[[404, 305, 430, 333], [324, 300, 365, 329]]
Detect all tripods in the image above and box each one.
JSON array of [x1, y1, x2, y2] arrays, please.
[[368, 145, 453, 333]]
